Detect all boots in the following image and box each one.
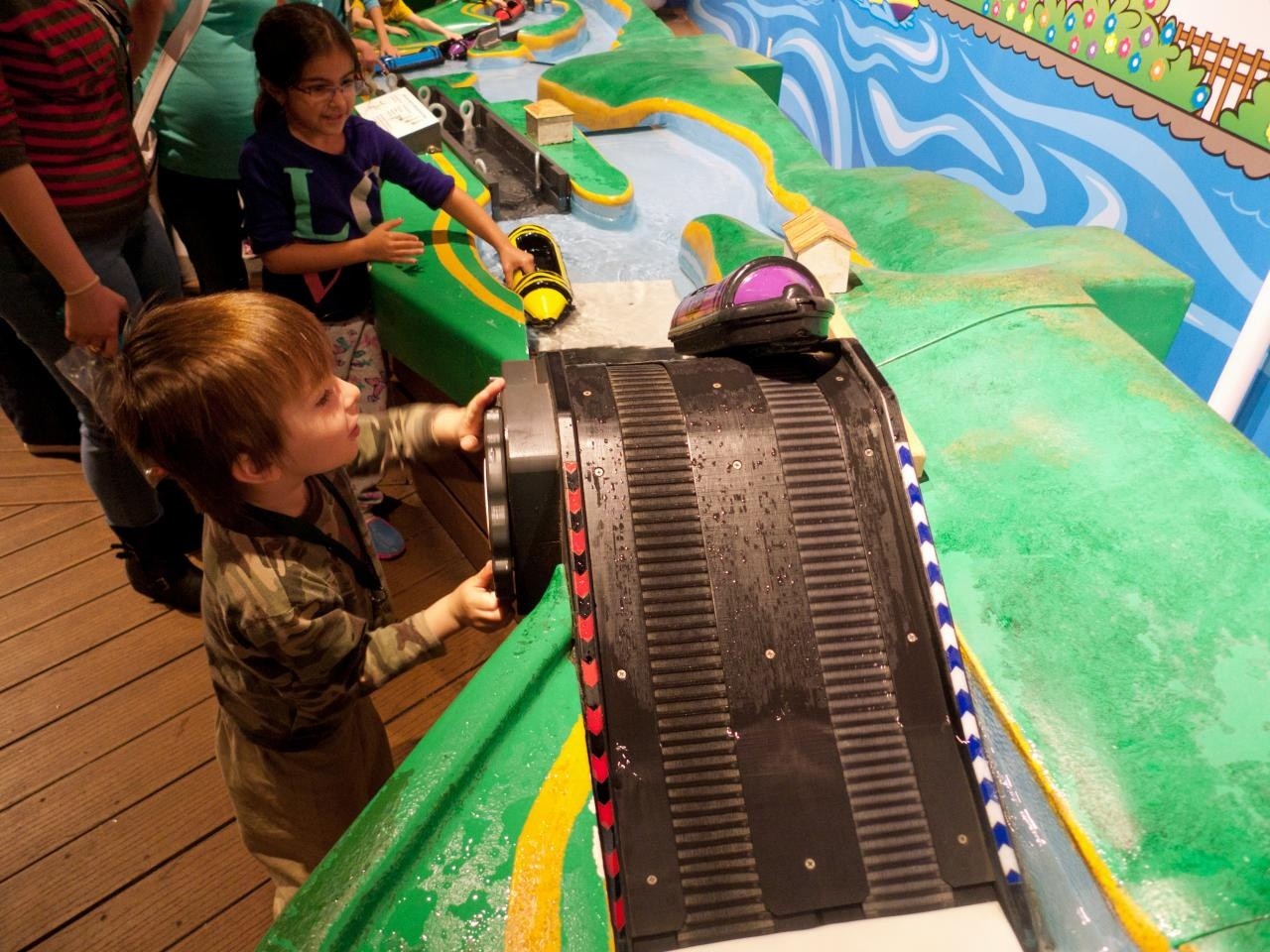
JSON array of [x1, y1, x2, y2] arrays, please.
[[109, 514, 203, 613]]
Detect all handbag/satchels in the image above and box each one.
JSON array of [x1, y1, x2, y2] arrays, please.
[[129, 122, 157, 197]]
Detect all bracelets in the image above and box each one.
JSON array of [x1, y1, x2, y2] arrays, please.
[[63, 276, 102, 300]]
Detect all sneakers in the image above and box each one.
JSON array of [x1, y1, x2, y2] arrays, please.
[[363, 509, 405, 559]]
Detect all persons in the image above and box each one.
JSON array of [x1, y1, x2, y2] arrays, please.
[[123, 0, 474, 294], [238, 1, 536, 570], [0, 0, 244, 614], [92, 291, 516, 925]]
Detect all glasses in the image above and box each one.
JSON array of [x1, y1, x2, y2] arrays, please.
[[293, 70, 364, 99]]
